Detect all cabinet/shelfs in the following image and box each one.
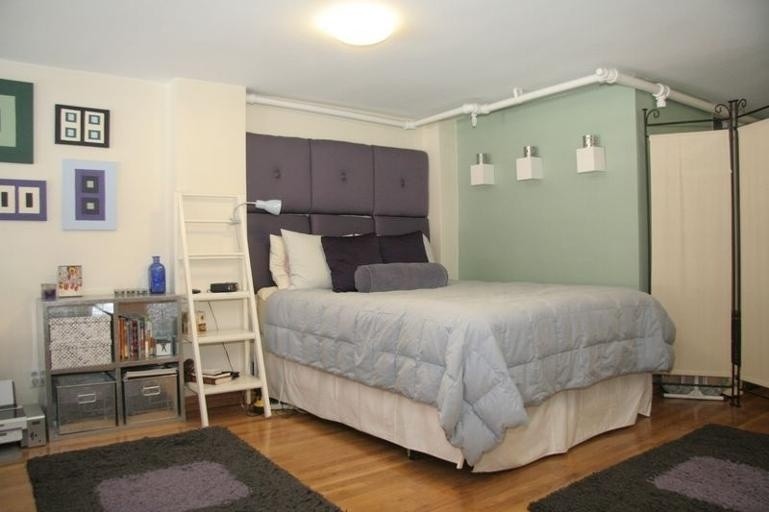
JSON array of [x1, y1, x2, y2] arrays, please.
[[175, 190, 271, 428], [36, 294, 185, 441]]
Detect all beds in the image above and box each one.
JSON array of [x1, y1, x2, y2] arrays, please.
[[253, 284, 677, 476]]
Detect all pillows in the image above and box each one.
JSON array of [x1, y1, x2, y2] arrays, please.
[[279, 228, 354, 290], [354, 233, 437, 262], [321, 232, 384, 292], [379, 230, 429, 263], [354, 262, 450, 292], [269, 234, 291, 291]]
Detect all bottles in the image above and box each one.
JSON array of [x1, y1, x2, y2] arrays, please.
[[149, 256, 166, 293]]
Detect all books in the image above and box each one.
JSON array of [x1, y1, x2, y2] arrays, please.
[[191, 369, 233, 386], [118, 312, 153, 362]]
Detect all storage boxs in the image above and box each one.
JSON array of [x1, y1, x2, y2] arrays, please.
[[53, 373, 116, 437], [124, 370, 177, 424], [49, 307, 112, 371]]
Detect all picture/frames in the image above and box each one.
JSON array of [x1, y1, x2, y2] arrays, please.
[[60, 160, 119, 230], [0, 79, 34, 164], [54, 104, 111, 148], [0, 179, 47, 221]]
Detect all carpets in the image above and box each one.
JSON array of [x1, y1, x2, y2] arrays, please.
[[25, 425, 342, 511], [526, 422, 769, 510]]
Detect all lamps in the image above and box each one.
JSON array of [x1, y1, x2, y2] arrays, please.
[[233, 199, 282, 244]]
